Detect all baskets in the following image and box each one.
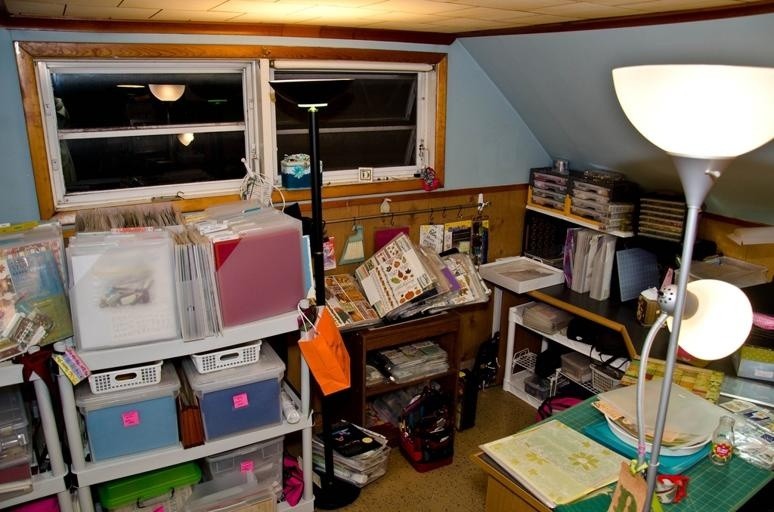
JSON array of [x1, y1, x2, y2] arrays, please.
[[86, 362, 165, 395], [590, 364, 620, 393], [189, 339, 261, 374]]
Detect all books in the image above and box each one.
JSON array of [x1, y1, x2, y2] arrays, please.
[[560, 226, 617, 302], [521, 301, 572, 336], [311, 418, 393, 489], [322, 230, 492, 334], [380, 340, 451, 386], [0, 463, 34, 501]]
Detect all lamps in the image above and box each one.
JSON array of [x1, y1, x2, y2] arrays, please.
[[609, 61, 772, 512]]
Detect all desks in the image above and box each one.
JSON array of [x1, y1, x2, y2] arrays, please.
[[470, 251, 773, 512]]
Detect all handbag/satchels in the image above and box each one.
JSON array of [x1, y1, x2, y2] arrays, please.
[[291, 301, 353, 397], [177, 384, 206, 452]]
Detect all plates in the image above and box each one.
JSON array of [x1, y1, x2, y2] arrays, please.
[[603, 414, 712, 457]]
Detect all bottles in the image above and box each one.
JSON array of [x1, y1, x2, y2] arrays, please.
[[710, 415, 736, 466]]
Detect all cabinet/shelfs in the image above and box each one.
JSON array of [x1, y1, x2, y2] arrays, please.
[[500, 296, 630, 416], [333, 311, 461, 474]]
